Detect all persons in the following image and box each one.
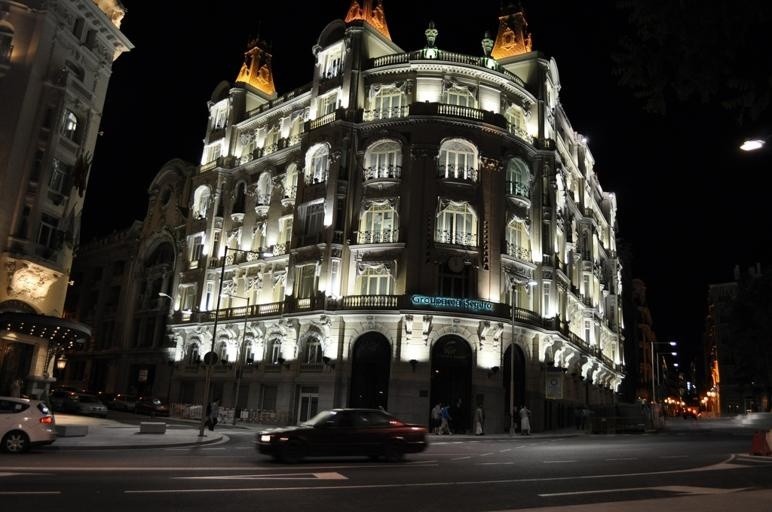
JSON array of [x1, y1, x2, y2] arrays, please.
[[211, 398, 220, 428], [431, 401, 532, 436]]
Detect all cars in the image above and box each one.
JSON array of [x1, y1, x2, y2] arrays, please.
[[51, 381, 172, 419], [252, 406, 432, 465], [682, 407, 698, 419]]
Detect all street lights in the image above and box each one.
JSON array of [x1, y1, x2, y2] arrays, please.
[[508, 279, 539, 437], [197, 244, 275, 436], [221, 291, 252, 427], [739, 135, 771, 154], [649, 338, 680, 428], [159, 291, 180, 400]]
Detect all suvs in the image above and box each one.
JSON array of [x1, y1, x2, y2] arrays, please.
[[1, 394, 58, 455]]
